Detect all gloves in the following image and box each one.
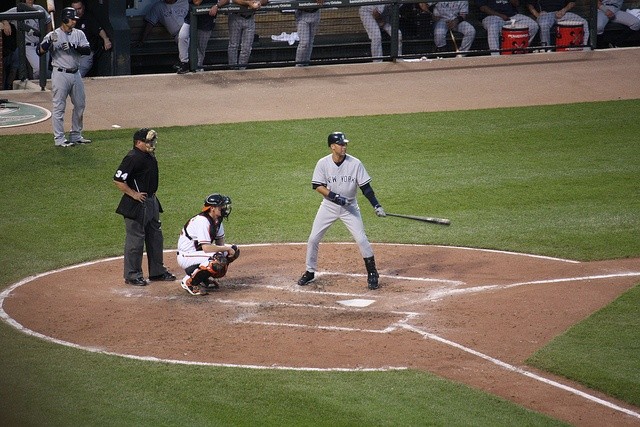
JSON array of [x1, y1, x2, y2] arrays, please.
[[47, 32, 57, 44], [333, 194, 355, 207], [372, 204, 385, 217], [61, 42, 77, 51]]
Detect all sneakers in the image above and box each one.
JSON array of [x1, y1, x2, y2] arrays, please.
[[180, 275, 206, 295], [75, 136, 92, 145], [176, 62, 189, 74], [200, 277, 220, 288], [124, 276, 148, 286], [297, 271, 315, 286], [149, 270, 176, 281], [55, 139, 75, 147], [366, 269, 379, 288]]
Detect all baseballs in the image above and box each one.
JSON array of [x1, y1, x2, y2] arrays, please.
[[46, 0, 56, 34]]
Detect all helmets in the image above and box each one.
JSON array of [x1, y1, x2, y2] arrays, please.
[[60, 7, 79, 23], [202, 192, 232, 218], [133, 128, 157, 154], [327, 132, 349, 147]]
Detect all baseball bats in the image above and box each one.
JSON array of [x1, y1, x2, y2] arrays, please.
[[386, 212, 451, 225], [449, 30, 462, 57]]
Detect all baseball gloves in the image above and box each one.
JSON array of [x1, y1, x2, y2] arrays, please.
[[225, 244, 240, 263]]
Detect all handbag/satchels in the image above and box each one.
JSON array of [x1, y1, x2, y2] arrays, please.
[[114, 180, 143, 221]]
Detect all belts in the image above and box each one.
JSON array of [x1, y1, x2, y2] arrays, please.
[[25, 42, 40, 46], [234, 12, 255, 19], [56, 68, 78, 74]]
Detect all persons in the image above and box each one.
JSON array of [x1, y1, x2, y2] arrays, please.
[[358, 1, 403, 64], [417, 0, 476, 57], [113, 127, 176, 286], [296, 2, 320, 67], [227, 1, 262, 69], [479, 1, 539, 56], [595, 1, 640, 42], [176, 194, 238, 296], [37, 8, 92, 149], [142, 1, 188, 40], [177, 0, 227, 74], [527, 1, 589, 51], [65, 2, 113, 75], [1, 0, 53, 79], [298, 132, 385, 289]]
